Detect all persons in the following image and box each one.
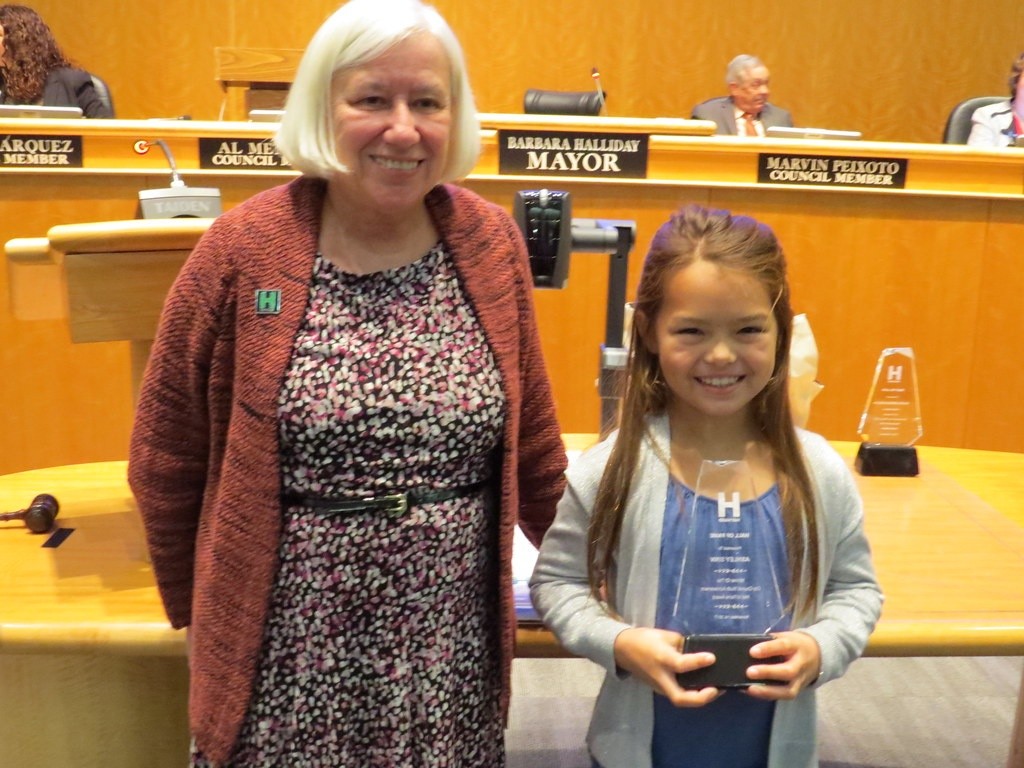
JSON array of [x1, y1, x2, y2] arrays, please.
[[124, 0, 570, 768], [0, 4, 114, 121], [693, 52, 795, 138], [531, 202, 887, 768], [966, 50, 1024, 152]]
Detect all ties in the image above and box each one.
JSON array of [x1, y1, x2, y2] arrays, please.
[[743, 113, 757, 136]]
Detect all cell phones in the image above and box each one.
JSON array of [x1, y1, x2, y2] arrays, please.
[[680, 632, 787, 690]]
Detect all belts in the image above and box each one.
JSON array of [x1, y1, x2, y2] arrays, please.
[[283, 480, 487, 519]]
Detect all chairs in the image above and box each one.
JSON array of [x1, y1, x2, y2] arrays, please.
[[943, 96, 1011, 141], [523, 89, 606, 114]]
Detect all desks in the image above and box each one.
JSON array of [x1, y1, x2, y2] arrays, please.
[[0, 433, 1024, 768]]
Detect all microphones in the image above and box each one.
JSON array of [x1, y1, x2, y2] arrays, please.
[[591, 68, 608, 117], [134, 141, 222, 221]]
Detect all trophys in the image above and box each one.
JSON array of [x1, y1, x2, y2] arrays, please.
[[676, 456, 796, 691], [856, 346, 925, 478]]
